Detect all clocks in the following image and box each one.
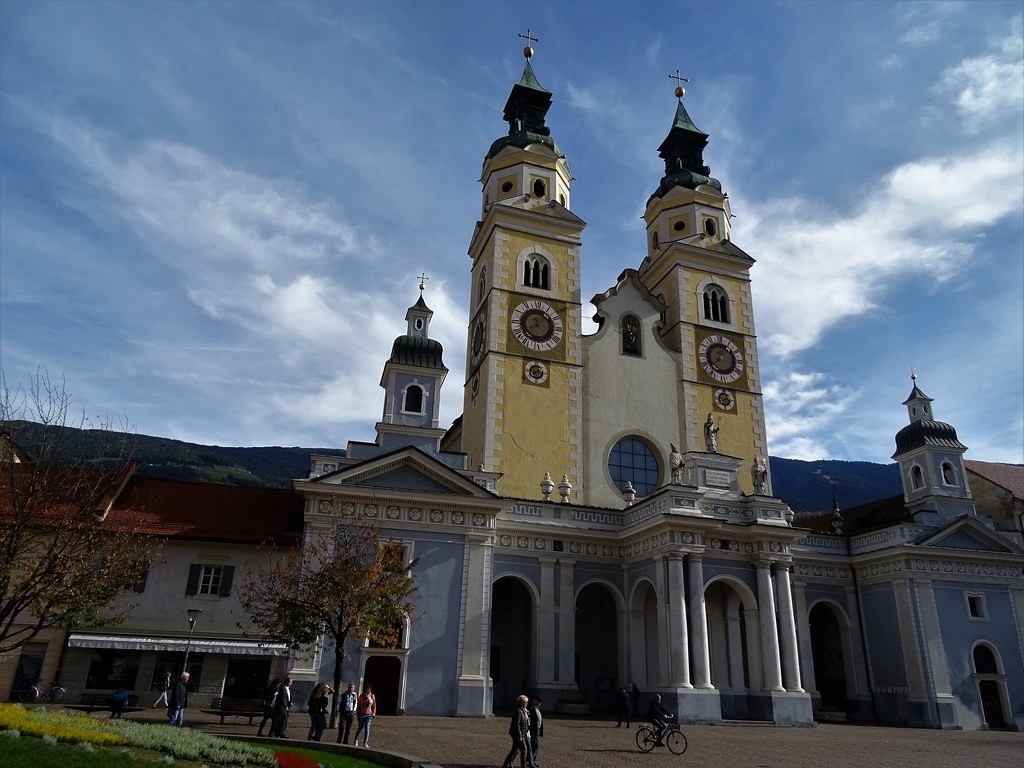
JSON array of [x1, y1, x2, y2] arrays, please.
[[511, 300, 563, 351], [714, 389, 734, 410], [471, 313, 486, 367], [525, 361, 548, 384], [698, 335, 743, 383]]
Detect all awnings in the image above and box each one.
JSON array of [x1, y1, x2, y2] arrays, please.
[[68, 633, 288, 656]]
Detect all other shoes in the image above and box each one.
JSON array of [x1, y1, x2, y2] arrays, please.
[[355, 740, 358, 747], [654, 731, 662, 738], [626, 726, 629, 728], [256, 733, 266, 737], [529, 764, 537, 768], [534, 761, 540, 767], [502, 764, 513, 768], [274, 732, 290, 739], [615, 724, 620, 727], [656, 742, 666, 747], [268, 732, 274, 737], [362, 744, 369, 748]]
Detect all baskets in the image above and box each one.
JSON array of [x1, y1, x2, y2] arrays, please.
[[670, 719, 680, 729]]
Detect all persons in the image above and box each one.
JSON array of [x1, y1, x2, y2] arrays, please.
[[109, 687, 128, 719], [308, 682, 335, 742], [704, 413, 717, 452], [751, 457, 765, 492], [502, 695, 543, 768], [616, 688, 629, 728], [670, 443, 685, 482], [647, 694, 673, 747], [152, 672, 171, 708], [355, 684, 376, 748], [257, 678, 293, 738], [168, 672, 190, 726], [335, 684, 358, 745]]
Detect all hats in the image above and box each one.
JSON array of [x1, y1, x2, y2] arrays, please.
[[533, 695, 543, 701]]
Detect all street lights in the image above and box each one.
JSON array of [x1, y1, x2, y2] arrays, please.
[[166, 607, 204, 718]]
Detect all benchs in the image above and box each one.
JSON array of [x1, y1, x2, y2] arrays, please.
[[201, 697, 266, 725], [62, 693, 145, 719]]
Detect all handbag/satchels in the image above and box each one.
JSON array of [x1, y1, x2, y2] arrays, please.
[[263, 686, 278, 708]]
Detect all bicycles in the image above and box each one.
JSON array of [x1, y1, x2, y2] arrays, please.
[[634, 713, 688, 755], [18, 678, 66, 704]]
